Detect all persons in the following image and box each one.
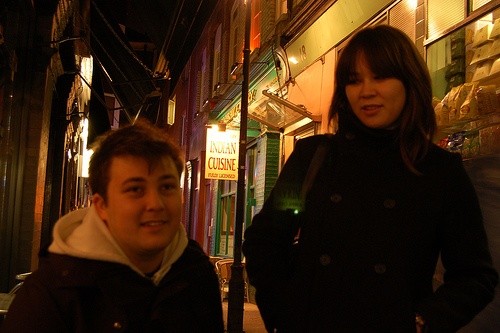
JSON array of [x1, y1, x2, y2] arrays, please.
[[2, 124, 224, 333], [242, 25, 500, 333]]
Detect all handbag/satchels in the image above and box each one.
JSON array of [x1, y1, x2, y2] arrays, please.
[[275, 241, 318, 333]]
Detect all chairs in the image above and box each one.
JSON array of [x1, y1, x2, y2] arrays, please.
[[215, 259, 250, 303]]
